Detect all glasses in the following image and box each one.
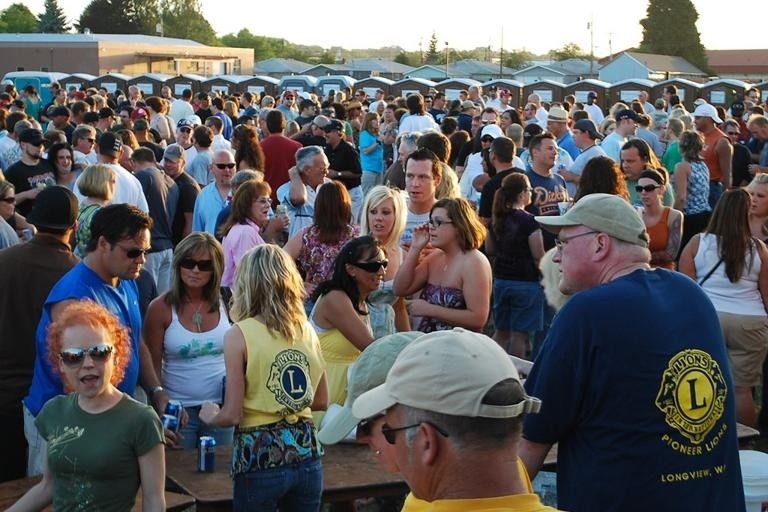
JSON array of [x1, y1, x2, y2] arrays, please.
[[118, 246, 152, 258], [351, 259, 388, 273], [60, 344, 113, 369], [180, 258, 214, 271]]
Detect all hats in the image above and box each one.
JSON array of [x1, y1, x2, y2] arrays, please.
[[317, 325, 543, 446], [18, 86, 744, 246]]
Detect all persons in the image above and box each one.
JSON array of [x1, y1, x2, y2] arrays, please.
[[2, 297, 173, 511], [354, 323, 559, 510], [0, 78, 768, 481], [190, 244, 330, 510], [519, 191, 749, 510]]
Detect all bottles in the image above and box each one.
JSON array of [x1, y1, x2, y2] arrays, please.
[[226, 192, 232, 204]]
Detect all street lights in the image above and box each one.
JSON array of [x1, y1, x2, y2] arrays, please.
[[444, 40, 449, 78], [585, 17, 597, 79]]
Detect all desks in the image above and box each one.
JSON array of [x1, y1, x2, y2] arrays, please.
[[161, 416, 759, 512], [0, 473, 198, 511]]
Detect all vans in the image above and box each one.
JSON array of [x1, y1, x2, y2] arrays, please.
[[1, 70, 62, 93]]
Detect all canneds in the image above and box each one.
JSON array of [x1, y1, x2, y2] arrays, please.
[[165, 399, 182, 435], [276, 205, 289, 229], [22, 228, 33, 241], [160, 414, 177, 434], [198, 437, 216, 472]]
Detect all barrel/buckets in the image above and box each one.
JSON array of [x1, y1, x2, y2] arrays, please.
[[738, 449, 768, 512]]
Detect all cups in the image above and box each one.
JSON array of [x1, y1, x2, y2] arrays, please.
[[558, 201, 573, 214]]
